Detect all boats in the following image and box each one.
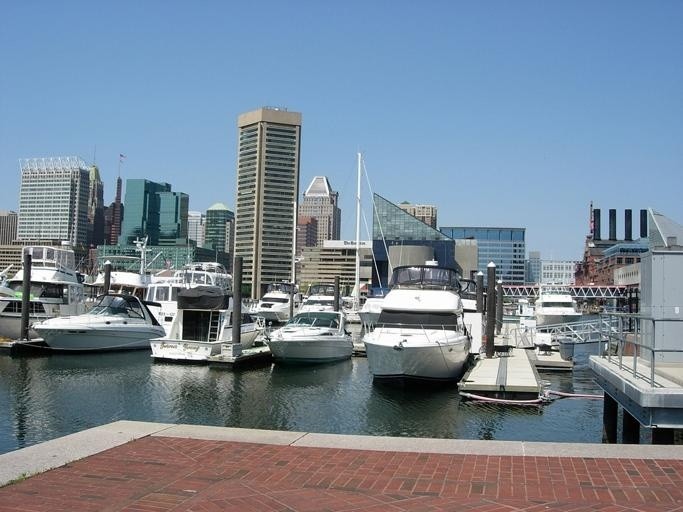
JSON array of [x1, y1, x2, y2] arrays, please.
[[535, 293, 582, 325]]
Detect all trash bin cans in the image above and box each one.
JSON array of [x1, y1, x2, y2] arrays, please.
[[558, 338, 574, 360]]
